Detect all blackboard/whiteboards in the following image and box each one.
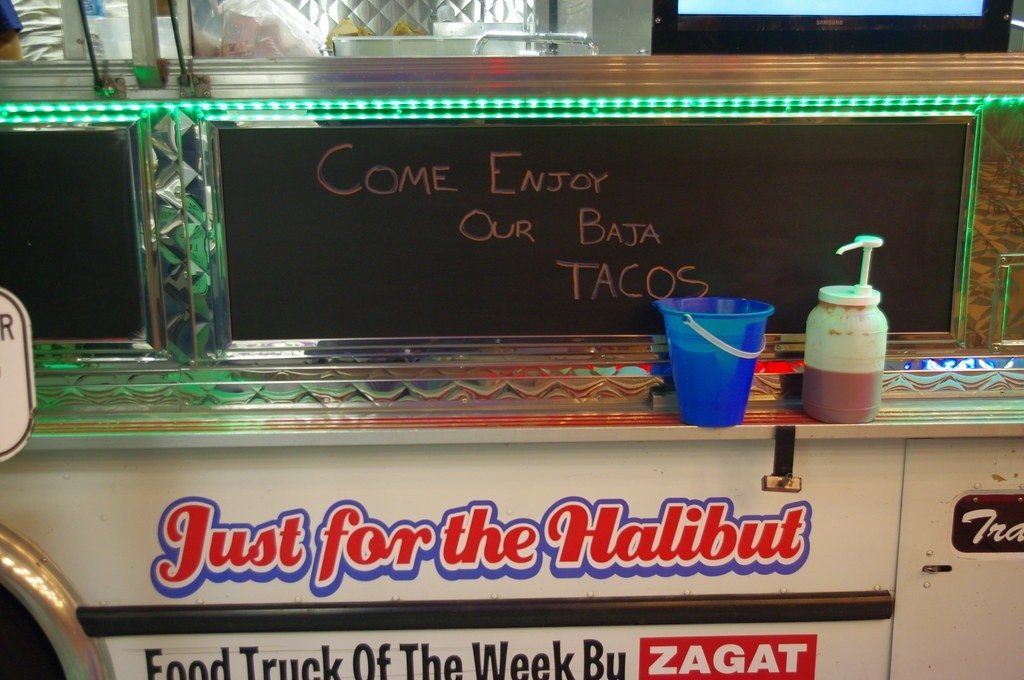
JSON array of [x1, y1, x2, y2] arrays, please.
[[199, 110, 983, 350], [1, 118, 163, 356]]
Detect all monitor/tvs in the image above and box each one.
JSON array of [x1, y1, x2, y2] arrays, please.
[[650, 0, 1013, 52]]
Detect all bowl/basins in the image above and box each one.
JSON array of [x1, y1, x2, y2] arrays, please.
[[96, 17, 178, 59]]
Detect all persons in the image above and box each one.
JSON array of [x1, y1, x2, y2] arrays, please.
[[190, 0, 327, 57], [0, 0, 24, 61]]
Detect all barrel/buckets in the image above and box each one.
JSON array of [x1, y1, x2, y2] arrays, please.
[[656, 298, 774, 427]]
